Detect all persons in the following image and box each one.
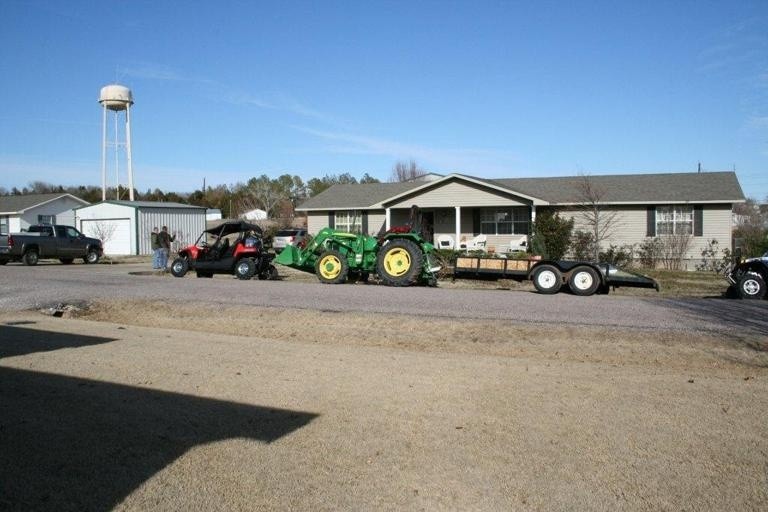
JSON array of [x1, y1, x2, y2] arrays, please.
[[158, 227, 176, 271], [152, 227, 160, 269]]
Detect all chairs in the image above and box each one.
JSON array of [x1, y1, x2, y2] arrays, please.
[[391, 204, 419, 234], [507, 235, 529, 252], [218, 237, 229, 255], [437, 233, 487, 254]]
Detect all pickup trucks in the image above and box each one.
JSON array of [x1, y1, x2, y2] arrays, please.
[[1, 224, 102, 265]]
